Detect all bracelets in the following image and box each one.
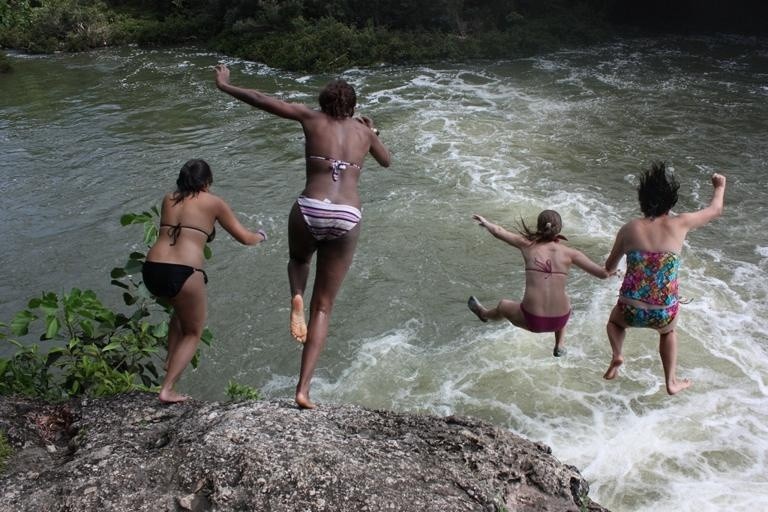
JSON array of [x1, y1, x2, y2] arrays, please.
[[259, 232, 265, 242], [371, 128, 379, 136]]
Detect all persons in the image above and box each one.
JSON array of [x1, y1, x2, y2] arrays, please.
[[468, 211, 620, 357], [606, 161, 726, 395], [143, 158, 268, 403], [213, 64, 391, 409]]
